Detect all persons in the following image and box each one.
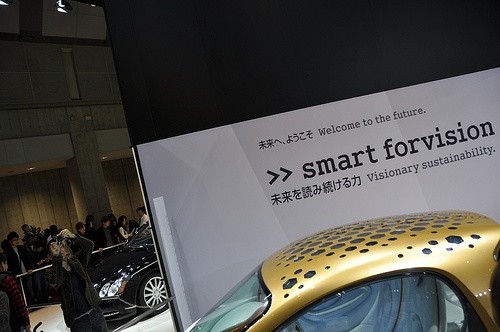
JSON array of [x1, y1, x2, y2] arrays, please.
[[125, 216, 140, 232], [137, 206, 148, 227], [4, 231, 35, 303], [20, 224, 36, 240], [50, 224, 60, 236], [84, 216, 99, 231], [0, 253, 31, 332], [25, 232, 43, 266], [47, 228, 111, 332], [43, 227, 51, 240], [118, 216, 135, 241], [108, 213, 116, 226], [76, 221, 103, 253], [0, 291, 13, 332], [99, 216, 126, 252]]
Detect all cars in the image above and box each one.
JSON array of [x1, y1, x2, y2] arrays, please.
[[176, 209, 500, 331], [83, 219, 170, 322]]
